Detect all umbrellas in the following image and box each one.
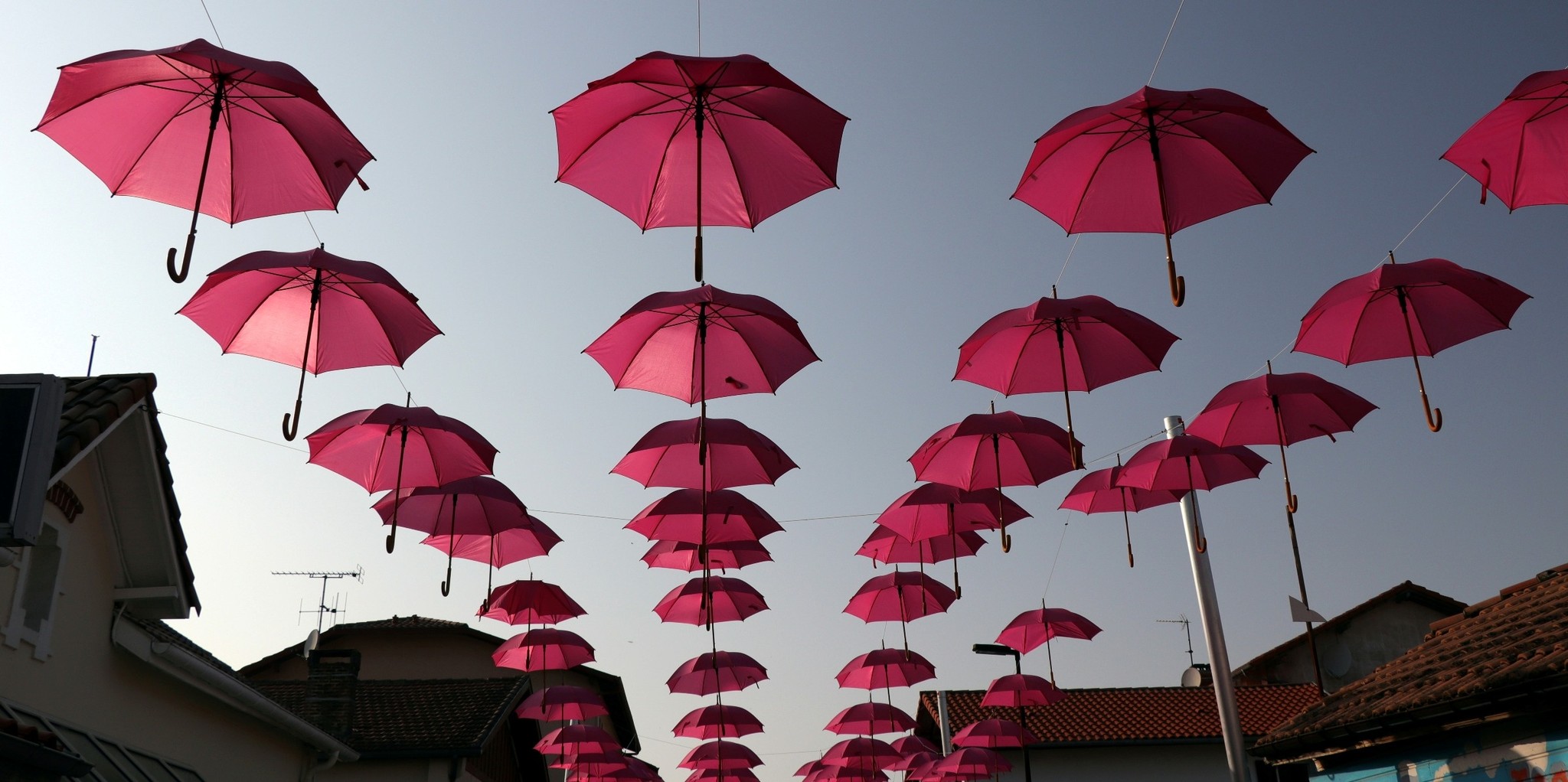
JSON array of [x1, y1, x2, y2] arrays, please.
[[305, 361, 1381, 782], [951, 283, 1181, 471], [31, 38, 377, 284], [547, 51, 850, 283], [174, 243, 446, 441], [581, 278, 823, 465], [1289, 250, 1534, 433], [1438, 66, 1567, 215], [1008, 83, 1318, 306]]
